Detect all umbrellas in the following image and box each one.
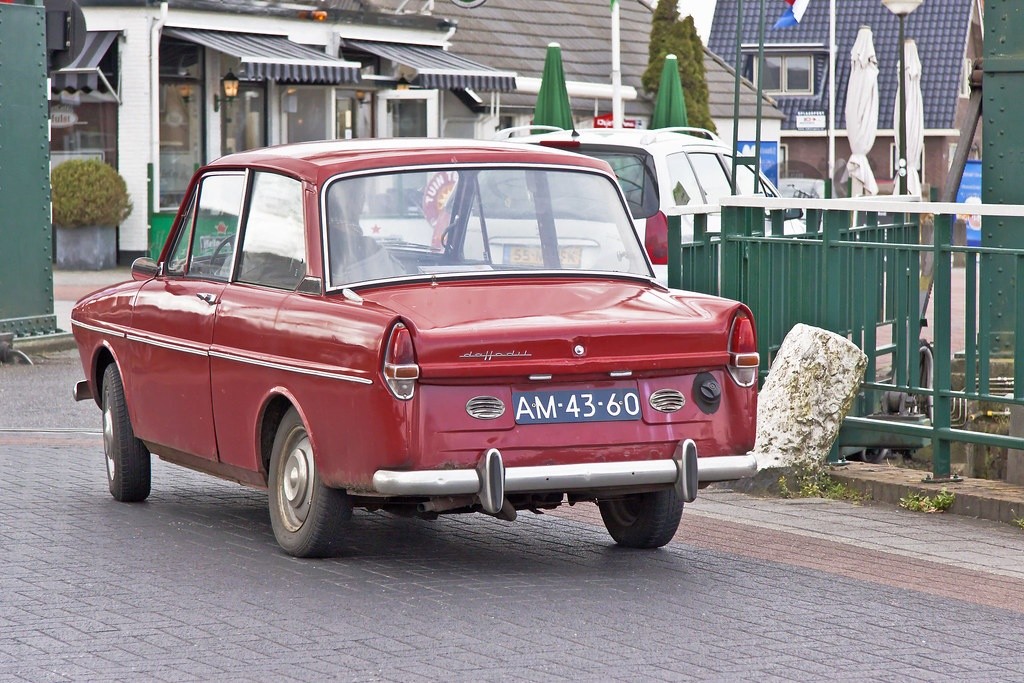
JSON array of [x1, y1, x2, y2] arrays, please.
[[892, 36, 925, 202], [844, 24, 880, 235], [530, 42, 576, 135], [649, 54, 690, 136]]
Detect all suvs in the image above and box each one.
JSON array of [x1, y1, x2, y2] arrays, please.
[[459, 125, 806, 291]]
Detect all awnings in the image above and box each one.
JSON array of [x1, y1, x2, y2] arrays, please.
[[340, 40, 518, 92], [50, 32, 122, 104], [160, 28, 362, 85]]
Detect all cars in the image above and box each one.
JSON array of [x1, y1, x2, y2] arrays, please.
[[69, 135, 763, 556]]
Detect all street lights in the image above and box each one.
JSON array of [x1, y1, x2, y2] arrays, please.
[[878, 0, 925, 195]]
